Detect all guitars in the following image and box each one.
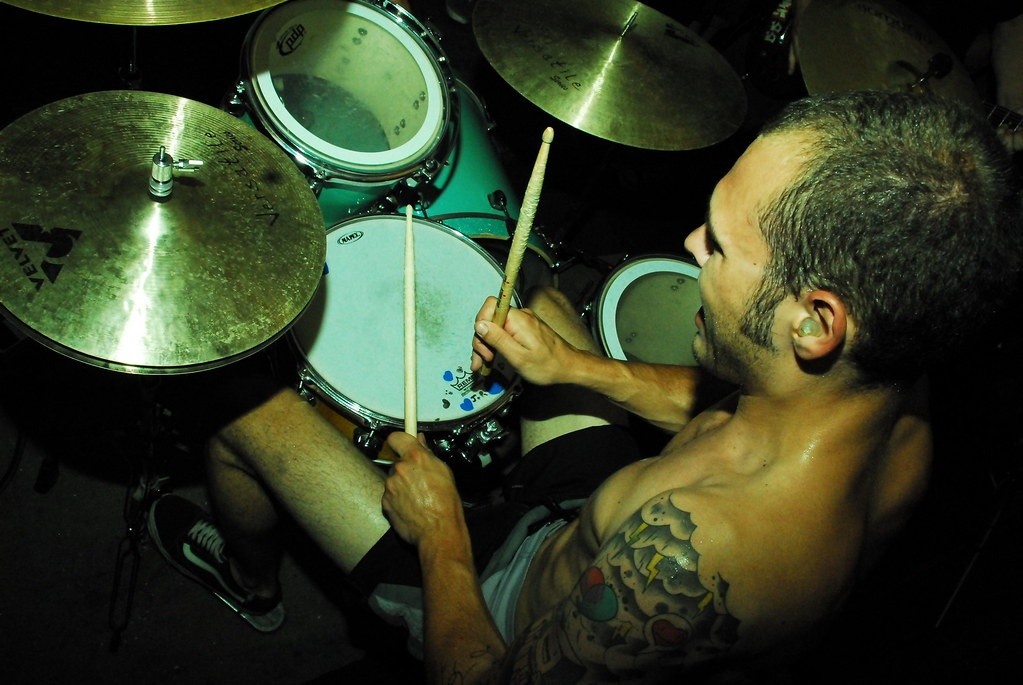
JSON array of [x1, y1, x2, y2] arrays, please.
[[979, 98, 1023, 135]]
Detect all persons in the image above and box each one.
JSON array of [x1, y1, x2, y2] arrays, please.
[[786, 0, 1023, 155], [146, 92, 1023, 685]]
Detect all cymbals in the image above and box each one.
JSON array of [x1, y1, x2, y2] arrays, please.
[[0, 0, 291, 27], [470, 0, 749, 152], [0, 88, 327, 376]]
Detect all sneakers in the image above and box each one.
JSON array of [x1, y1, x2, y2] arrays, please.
[[150, 494, 283, 618]]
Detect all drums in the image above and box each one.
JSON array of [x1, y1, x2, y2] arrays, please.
[[220, 70, 561, 299], [220, 0, 462, 229], [578, 250, 705, 366], [271, 213, 522, 469]]
